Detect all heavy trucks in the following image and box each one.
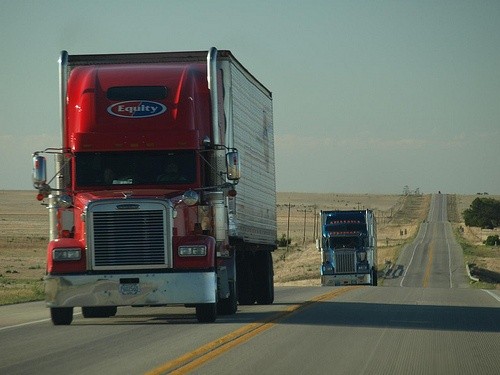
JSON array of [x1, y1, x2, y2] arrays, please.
[[315, 209, 378, 288], [29, 48, 279, 324]]
